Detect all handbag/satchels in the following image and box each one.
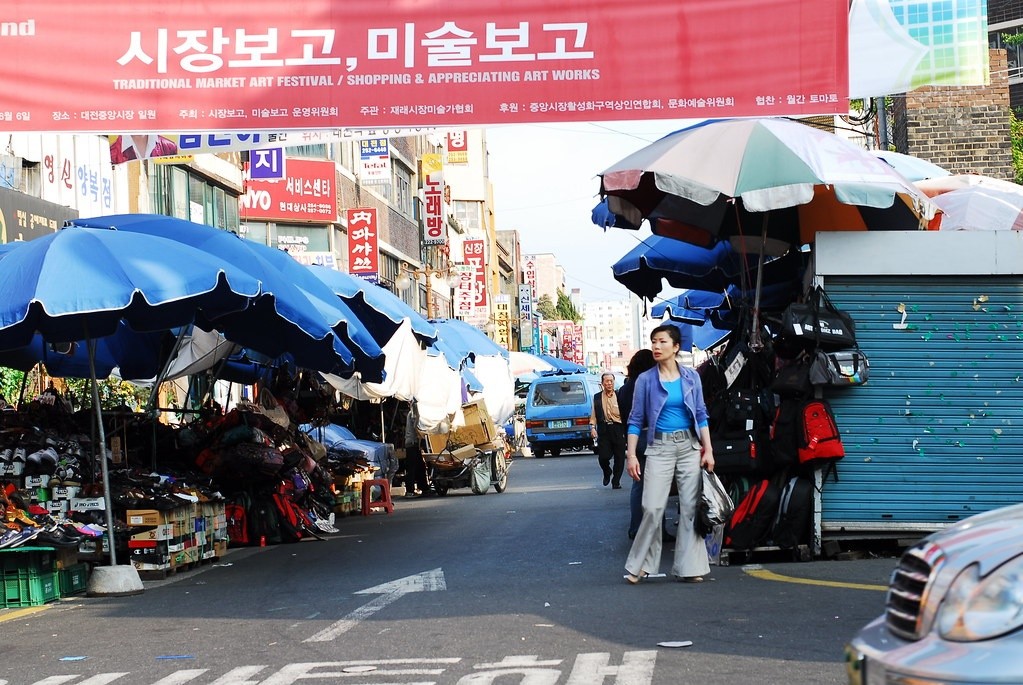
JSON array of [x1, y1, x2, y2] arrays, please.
[[696, 285, 870, 557], [175, 361, 340, 546]]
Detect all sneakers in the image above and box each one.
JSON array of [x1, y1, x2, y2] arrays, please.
[[405, 492, 421, 498], [0, 423, 225, 548], [422, 490, 435, 496]]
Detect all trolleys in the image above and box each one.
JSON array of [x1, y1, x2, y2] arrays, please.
[[431, 450, 515, 496]]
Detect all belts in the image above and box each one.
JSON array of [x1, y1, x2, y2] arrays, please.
[[606, 422, 621, 426], [655, 430, 697, 440]]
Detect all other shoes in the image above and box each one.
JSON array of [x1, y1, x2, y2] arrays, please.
[[662, 531, 676, 542], [628, 527, 638, 539]]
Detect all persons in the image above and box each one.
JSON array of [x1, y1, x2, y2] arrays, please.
[[589, 371, 626, 489], [109, 135, 177, 165], [617, 324, 715, 584]]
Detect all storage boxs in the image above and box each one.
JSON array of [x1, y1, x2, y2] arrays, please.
[[55, 548, 78, 568], [60, 568, 86, 598], [428, 399, 495, 461], [0, 573, 59, 608], [127, 502, 228, 571], [0, 546, 55, 573]]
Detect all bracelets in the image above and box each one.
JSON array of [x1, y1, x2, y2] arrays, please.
[[592, 425, 595, 429], [627, 455, 636, 459]]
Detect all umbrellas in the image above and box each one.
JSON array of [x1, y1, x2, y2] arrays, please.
[[509, 351, 586, 377], [592, 118, 1023, 352], [0, 213, 510, 567]]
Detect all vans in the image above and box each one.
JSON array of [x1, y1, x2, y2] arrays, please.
[[524, 375, 620, 458]]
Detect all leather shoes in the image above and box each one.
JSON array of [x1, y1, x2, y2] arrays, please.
[[603, 469, 612, 486], [613, 485, 621, 489]]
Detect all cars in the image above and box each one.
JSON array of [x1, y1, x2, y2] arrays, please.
[[844, 503, 1022, 685]]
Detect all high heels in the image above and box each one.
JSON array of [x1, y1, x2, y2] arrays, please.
[[628, 570, 649, 583], [675, 575, 703, 583]]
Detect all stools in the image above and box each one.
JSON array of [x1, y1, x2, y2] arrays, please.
[[362, 479, 394, 515]]
